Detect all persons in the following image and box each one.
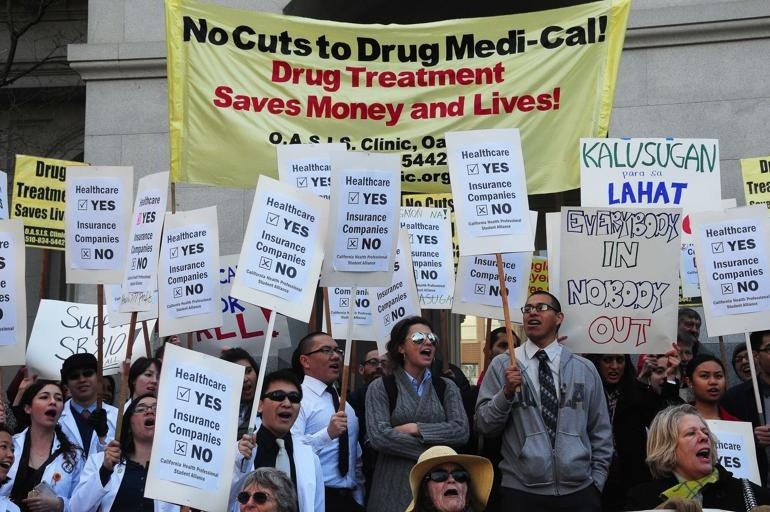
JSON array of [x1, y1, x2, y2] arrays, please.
[[230, 371, 325, 512], [477, 327, 520, 385], [690, 355, 742, 421], [556, 335, 682, 512], [366, 315, 471, 511], [732, 343, 759, 381], [240, 467, 298, 512], [676, 342, 693, 374], [0, 382, 84, 512], [123, 358, 161, 415], [473, 291, 614, 511], [721, 329, 770, 512], [0, 430, 22, 512], [637, 355, 681, 394], [678, 308, 713, 357], [646, 404, 748, 512], [59, 354, 118, 498], [68, 393, 190, 512], [219, 348, 259, 440], [291, 332, 368, 512], [348, 349, 390, 417], [0, 373, 39, 433]]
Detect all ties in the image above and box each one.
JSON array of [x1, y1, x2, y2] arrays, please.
[[534, 350, 559, 450], [274, 438, 291, 480], [325, 386, 349, 477]]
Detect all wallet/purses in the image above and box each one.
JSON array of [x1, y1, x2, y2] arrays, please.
[[27, 480, 56, 500]]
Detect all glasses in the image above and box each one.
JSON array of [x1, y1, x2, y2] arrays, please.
[[364, 358, 386, 366], [411, 331, 440, 345], [69, 370, 96, 380], [261, 390, 301, 403], [424, 467, 471, 483], [133, 405, 156, 413], [236, 492, 269, 504], [520, 303, 559, 313], [759, 343, 770, 353], [303, 345, 344, 355]]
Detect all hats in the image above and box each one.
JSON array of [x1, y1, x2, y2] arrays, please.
[[62, 352, 97, 376]]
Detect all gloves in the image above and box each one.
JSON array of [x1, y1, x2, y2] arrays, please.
[[88, 408, 109, 437]]
